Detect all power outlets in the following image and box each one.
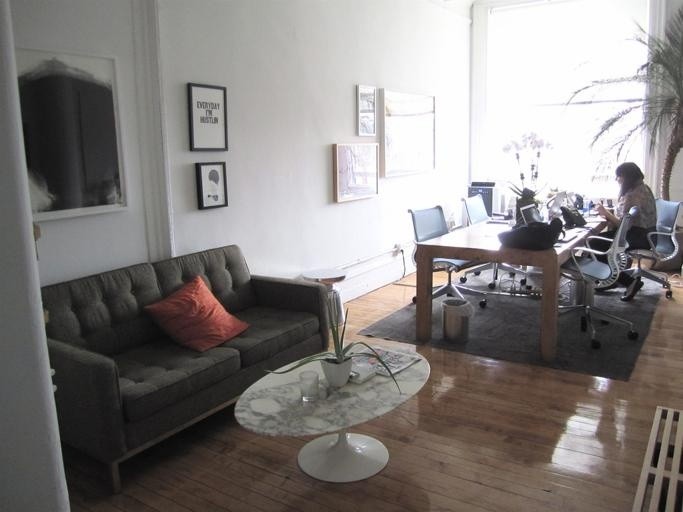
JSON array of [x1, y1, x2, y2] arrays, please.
[[395, 243, 401, 255]]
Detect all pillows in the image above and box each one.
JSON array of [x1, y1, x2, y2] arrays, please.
[[143, 275, 251, 353]]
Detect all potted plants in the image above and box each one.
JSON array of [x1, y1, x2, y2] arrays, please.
[[567, 7, 683, 272], [262, 292, 402, 396]]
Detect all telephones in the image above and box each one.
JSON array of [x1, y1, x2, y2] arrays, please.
[[559, 206, 588, 229]]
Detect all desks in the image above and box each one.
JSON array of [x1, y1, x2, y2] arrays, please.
[[415, 218, 608, 365]]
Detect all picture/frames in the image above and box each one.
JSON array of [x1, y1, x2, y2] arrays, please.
[[380, 88, 438, 180], [194, 162, 228, 209], [356, 84, 377, 138], [16, 47, 128, 223], [186, 83, 228, 152], [332, 143, 379, 203]]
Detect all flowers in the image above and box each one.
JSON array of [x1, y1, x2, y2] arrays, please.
[[508, 132, 554, 199]]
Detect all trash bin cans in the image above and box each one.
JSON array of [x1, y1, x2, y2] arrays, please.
[[441, 297, 474, 342]]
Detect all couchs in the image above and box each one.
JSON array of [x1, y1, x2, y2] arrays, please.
[[40, 244, 331, 493]]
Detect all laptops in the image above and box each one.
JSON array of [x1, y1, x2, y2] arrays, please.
[[518, 204, 577, 243]]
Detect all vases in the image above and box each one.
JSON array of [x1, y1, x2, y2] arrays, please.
[[516, 197, 538, 223]]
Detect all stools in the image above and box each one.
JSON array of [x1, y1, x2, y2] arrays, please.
[[303, 267, 347, 329]]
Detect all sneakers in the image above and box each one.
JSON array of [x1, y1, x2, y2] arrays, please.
[[621, 279, 644, 301]]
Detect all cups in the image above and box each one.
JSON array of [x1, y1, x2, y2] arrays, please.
[[299, 371, 319, 404]]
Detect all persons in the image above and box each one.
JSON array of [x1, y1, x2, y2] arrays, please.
[[583, 162, 659, 299]]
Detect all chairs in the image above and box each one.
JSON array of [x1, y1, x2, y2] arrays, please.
[[460, 193, 527, 289], [595, 198, 682, 299], [557, 206, 642, 353], [407, 205, 488, 314]]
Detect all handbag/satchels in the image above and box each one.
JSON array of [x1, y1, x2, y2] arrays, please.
[[547, 190, 574, 220], [560, 203, 587, 228], [497, 217, 566, 251]]
